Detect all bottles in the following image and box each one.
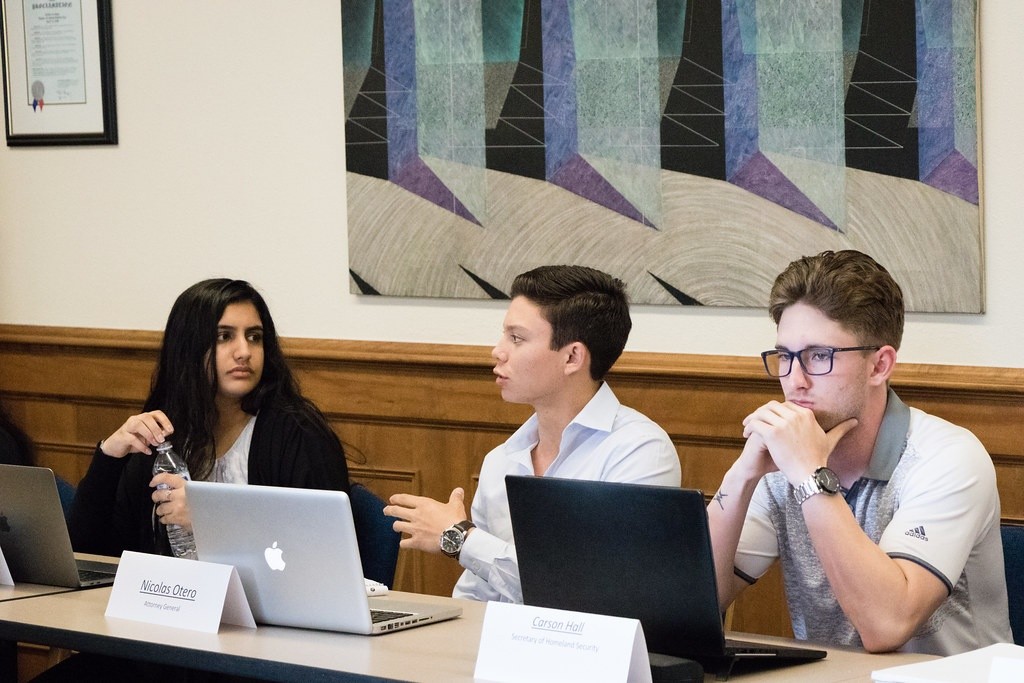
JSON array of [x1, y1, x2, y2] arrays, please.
[[152, 442, 199, 560]]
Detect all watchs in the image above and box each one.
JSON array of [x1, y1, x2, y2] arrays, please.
[[793, 466, 841, 506], [438, 519, 477, 560]]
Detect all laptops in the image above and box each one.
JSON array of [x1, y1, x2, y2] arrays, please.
[[185, 481, 462, 635], [0, 464, 118, 588], [505, 474, 828, 661]]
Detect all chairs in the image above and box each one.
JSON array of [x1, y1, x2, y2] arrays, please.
[[350, 481, 404, 589]]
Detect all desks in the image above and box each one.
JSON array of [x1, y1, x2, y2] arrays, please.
[[0, 554, 944, 683]]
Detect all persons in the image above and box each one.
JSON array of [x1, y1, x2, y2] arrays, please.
[[705, 248, 1016, 659], [25, 279, 351, 683], [381, 266, 681, 604]]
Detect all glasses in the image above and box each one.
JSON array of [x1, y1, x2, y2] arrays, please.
[[761, 346, 880, 377]]
